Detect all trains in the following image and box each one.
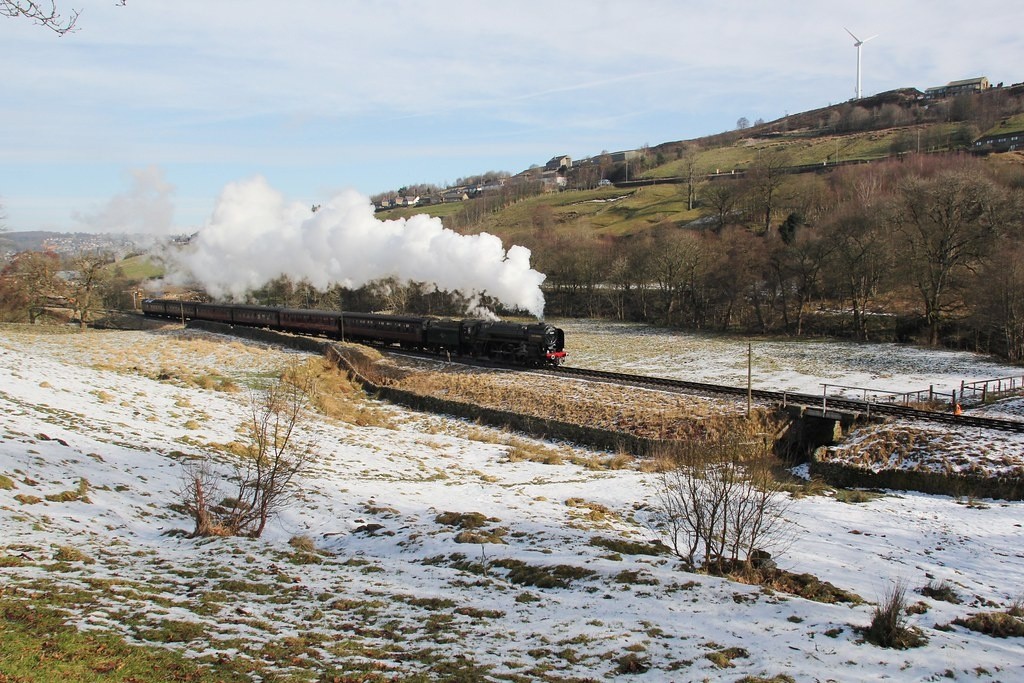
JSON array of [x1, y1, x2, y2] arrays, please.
[[142, 298, 569, 371]]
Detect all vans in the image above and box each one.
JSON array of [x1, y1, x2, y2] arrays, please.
[[598, 179, 613, 185]]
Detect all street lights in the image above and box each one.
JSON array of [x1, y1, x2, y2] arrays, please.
[[653, 164, 659, 185], [917, 129, 923, 154], [625, 163, 632, 182], [835, 137, 841, 165], [758, 149, 765, 175]]
